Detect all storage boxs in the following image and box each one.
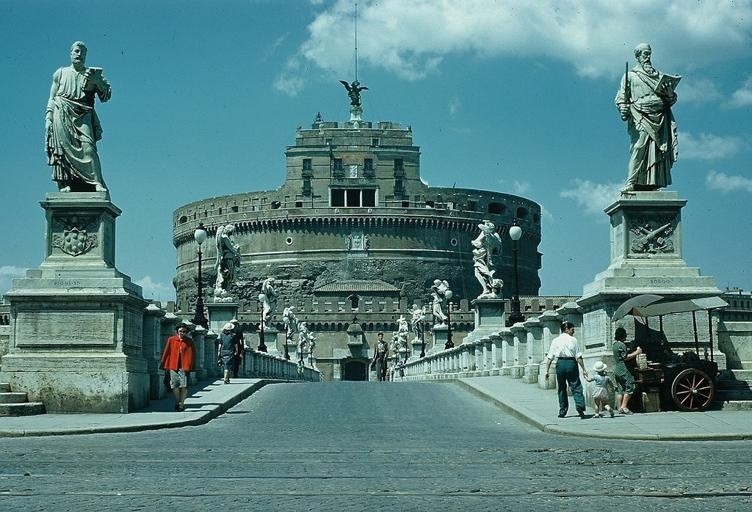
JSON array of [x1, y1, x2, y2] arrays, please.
[[640, 393, 661, 413]]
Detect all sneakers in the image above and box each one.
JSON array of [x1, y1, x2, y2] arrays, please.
[[593, 414, 599, 418], [618, 407, 634, 415], [576, 406, 585, 418], [609, 407, 614, 417], [558, 413, 565, 418]]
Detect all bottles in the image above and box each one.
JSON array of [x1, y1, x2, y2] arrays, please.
[[636, 354, 647, 369]]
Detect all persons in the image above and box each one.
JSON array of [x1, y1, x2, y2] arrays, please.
[[214, 223, 319, 357], [390, 280, 453, 357], [217, 322, 239, 383], [160, 323, 196, 411], [585, 361, 618, 418], [616, 42, 680, 190], [227, 318, 244, 377], [611, 328, 642, 413], [44, 41, 112, 191], [370, 332, 389, 381], [544, 321, 588, 417], [470, 218, 502, 297]]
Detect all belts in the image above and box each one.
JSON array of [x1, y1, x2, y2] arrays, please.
[[558, 357, 576, 360]]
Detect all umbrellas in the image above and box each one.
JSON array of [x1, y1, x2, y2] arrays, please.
[[610, 294, 665, 326]]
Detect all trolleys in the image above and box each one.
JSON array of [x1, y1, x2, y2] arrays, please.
[[630, 293, 731, 413]]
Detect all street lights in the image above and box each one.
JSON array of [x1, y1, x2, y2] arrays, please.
[[443, 287, 455, 350], [505, 217, 527, 326], [188, 223, 215, 330], [388, 328, 408, 366], [419, 309, 430, 358], [298, 337, 305, 365], [281, 312, 291, 360], [310, 338, 315, 369], [256, 290, 269, 353]]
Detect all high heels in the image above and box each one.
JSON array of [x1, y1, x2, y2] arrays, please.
[[223, 380, 230, 384], [174, 400, 185, 412]]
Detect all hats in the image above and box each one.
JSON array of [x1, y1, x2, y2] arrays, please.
[[222, 323, 235, 330], [593, 361, 607, 372], [175, 323, 190, 332], [229, 318, 239, 323]]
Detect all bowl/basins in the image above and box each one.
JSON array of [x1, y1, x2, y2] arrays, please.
[[647, 361, 663, 369]]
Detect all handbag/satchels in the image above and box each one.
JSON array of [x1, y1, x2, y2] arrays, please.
[[635, 346, 648, 369], [217, 355, 223, 368]]
